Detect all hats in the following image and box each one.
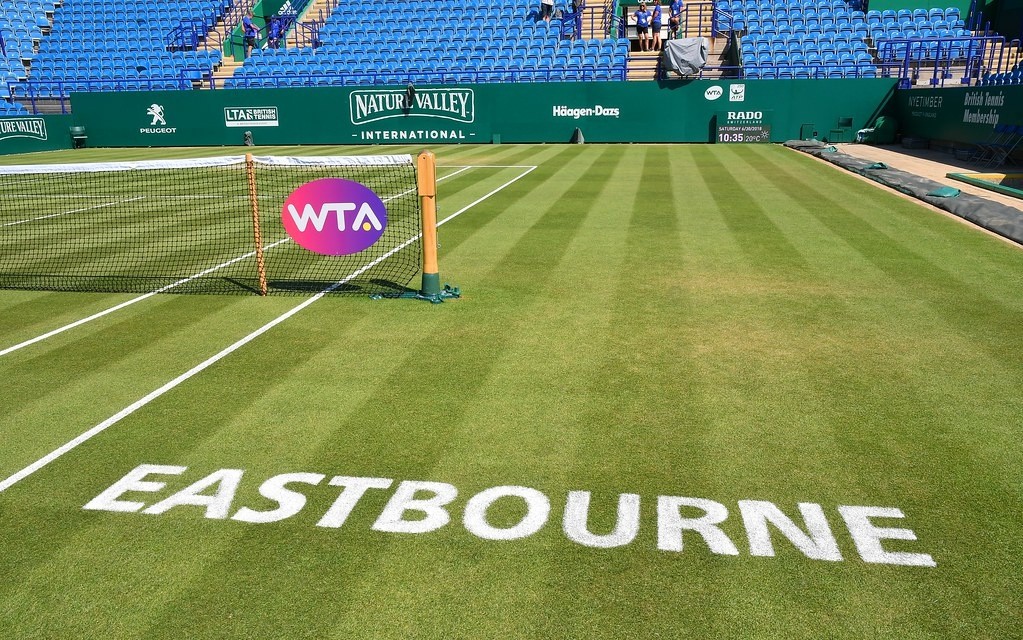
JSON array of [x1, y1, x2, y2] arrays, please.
[[271, 14, 276, 18]]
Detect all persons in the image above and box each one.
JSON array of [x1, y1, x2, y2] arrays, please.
[[631, 2, 654, 53], [541, 0, 554, 23], [270, 15, 284, 49], [649, 0, 662, 51], [668, 0, 685, 40], [242, 9, 260, 57], [571, 0, 583, 29]]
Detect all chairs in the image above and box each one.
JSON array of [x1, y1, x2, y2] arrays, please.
[[974, 124, 1020, 169], [829, 118, 853, 143], [714, 0, 1023, 86], [69, 125, 89, 149], [860, 114, 888, 145], [965, 123, 1008, 167], [0, 0, 631, 117], [986, 126, 1023, 170]]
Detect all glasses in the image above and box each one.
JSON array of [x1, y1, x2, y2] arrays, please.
[[639, 5, 645, 8]]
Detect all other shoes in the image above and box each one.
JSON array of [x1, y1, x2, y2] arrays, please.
[[650, 48, 655, 52], [640, 50, 644, 52], [644, 49, 650, 52], [656, 49, 661, 51]]
